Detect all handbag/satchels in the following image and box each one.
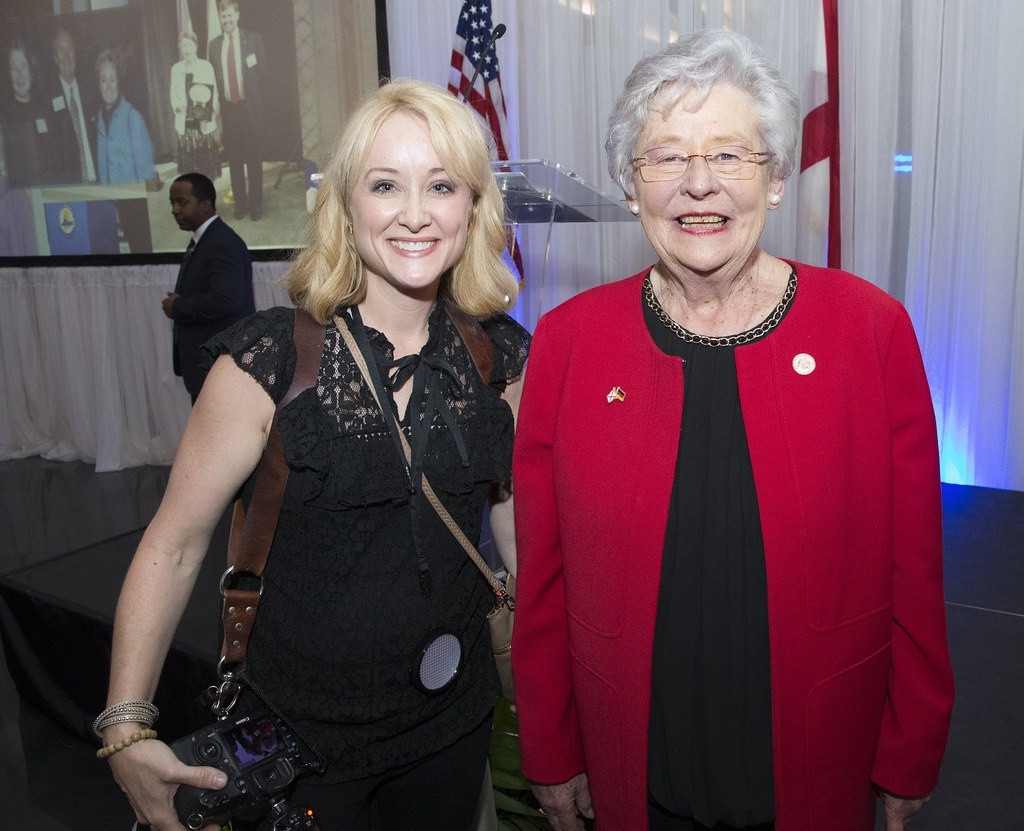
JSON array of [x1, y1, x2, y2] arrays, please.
[[487, 574, 516, 712]]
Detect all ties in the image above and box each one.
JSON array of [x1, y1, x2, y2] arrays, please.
[[181, 237, 197, 281], [227, 36, 240, 105], [69, 86, 89, 187]]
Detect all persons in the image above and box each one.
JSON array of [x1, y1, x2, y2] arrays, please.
[[92, 80, 531, 831], [162, 174, 258, 409], [42, 30, 99, 185], [92, 50, 157, 188], [0, 39, 62, 188], [235, 720, 278, 757], [206, 1, 274, 220], [511, 23, 954, 831], [167, 30, 224, 182]]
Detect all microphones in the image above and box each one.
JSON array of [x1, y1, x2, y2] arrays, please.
[[463, 24, 506, 103]]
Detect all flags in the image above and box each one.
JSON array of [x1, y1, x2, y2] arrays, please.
[[446, 0, 524, 282]]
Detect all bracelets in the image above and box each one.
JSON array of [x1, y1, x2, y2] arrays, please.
[[92, 700, 160, 738], [95, 730, 157, 758]]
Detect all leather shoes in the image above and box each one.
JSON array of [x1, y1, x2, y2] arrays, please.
[[249, 205, 261, 221], [232, 201, 247, 220]]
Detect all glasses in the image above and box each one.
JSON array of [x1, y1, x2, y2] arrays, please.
[[628, 144, 774, 183]]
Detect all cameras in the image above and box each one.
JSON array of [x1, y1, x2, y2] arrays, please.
[[167, 703, 327, 831]]
